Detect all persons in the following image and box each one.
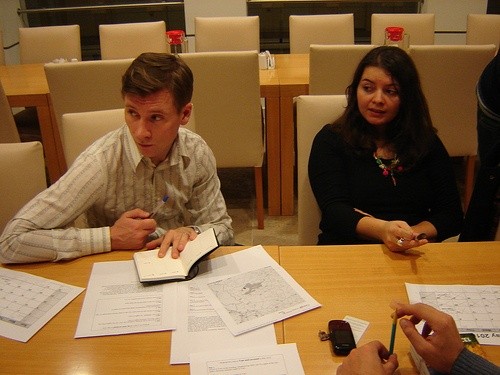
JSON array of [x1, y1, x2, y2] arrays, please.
[[336, 301, 500, 375], [458, 50, 500, 243], [308, 45, 464, 252], [0, 51, 243, 264]]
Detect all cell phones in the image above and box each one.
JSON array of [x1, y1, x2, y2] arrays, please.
[[328, 320, 357, 355]]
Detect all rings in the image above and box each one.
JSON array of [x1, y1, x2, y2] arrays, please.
[[396, 238, 404, 247], [184, 232, 188, 234]]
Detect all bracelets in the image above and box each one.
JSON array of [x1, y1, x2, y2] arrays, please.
[[191, 226, 199, 233]]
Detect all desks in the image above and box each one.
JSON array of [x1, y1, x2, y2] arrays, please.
[[0, 241, 500, 375], [0, 53, 280, 217], [280, 55, 309, 217]]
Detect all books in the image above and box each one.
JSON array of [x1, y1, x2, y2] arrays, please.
[[133, 227, 220, 283]]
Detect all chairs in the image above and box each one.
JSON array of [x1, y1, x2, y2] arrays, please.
[[0, 13, 499, 248]]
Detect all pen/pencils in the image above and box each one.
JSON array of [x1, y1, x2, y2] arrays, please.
[[147, 196, 168, 219], [412, 233, 427, 241], [388, 307, 397, 355]]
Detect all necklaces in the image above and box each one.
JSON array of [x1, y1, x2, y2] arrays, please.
[[374, 152, 403, 187]]
